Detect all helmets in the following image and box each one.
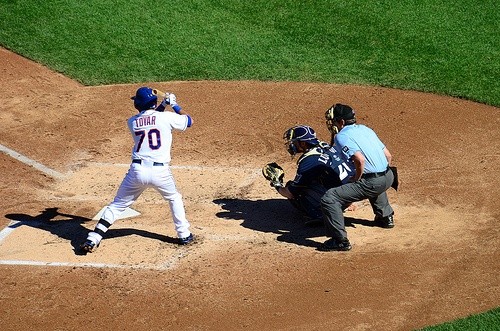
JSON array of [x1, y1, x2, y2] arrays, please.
[[131, 87, 157, 106]]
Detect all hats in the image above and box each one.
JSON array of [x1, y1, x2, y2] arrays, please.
[[293, 126, 321, 145]]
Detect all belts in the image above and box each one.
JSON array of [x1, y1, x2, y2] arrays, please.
[[361, 166, 390, 179], [132, 159, 164, 166]]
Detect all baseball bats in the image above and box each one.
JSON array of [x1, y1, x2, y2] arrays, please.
[[152, 89, 166, 98]]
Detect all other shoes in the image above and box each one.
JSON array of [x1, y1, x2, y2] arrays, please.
[[317, 239, 352, 251], [178, 233, 195, 248], [79, 237, 96, 253], [373, 216, 394, 228], [302, 213, 325, 227]]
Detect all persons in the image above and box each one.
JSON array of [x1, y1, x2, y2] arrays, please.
[[317, 104, 395, 251], [82, 87, 194, 253], [261, 125, 357, 228]]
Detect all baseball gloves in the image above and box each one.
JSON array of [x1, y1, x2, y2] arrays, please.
[[262, 162, 284, 187]]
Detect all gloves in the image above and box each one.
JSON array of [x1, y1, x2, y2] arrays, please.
[[160, 93, 178, 108]]
